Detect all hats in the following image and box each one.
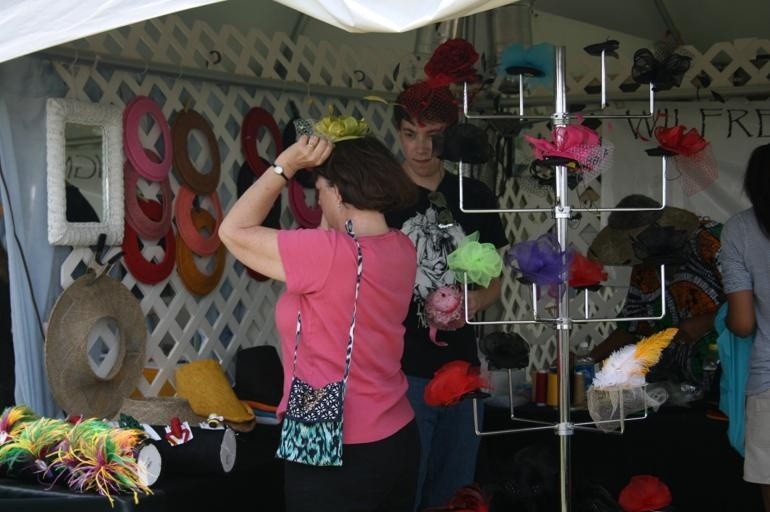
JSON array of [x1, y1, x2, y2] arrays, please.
[[119, 344, 284, 433], [45, 274, 147, 419]]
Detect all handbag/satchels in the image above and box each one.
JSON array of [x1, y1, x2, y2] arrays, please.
[[274, 378, 345, 467]]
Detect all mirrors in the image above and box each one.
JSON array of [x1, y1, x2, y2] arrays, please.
[[45, 97, 127, 248]]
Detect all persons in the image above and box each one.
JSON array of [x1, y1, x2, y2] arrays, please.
[[713, 143, 770, 511], [216, 114, 421, 512], [385, 77, 509, 512]]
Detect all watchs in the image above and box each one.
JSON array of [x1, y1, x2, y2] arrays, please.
[[270, 160, 291, 184]]
